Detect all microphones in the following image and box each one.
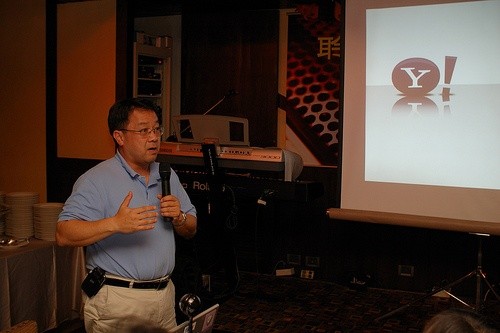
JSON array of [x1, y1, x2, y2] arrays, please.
[[159, 157, 173, 222]]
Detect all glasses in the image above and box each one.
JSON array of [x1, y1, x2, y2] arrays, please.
[[115, 126, 165, 137]]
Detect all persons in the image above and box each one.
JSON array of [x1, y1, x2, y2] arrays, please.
[[424, 309, 485, 333], [56, 99, 197, 333]]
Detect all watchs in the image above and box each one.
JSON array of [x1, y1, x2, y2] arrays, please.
[[174, 210, 187, 226]]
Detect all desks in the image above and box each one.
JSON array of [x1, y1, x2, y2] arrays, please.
[[0, 237, 86, 333]]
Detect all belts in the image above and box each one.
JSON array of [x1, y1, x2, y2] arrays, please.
[[103, 278, 169, 290]]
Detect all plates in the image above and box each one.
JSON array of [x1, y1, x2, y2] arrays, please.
[[0, 192, 64, 248]]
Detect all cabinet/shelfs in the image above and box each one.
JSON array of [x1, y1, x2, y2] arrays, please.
[[133, 42, 172, 141]]
